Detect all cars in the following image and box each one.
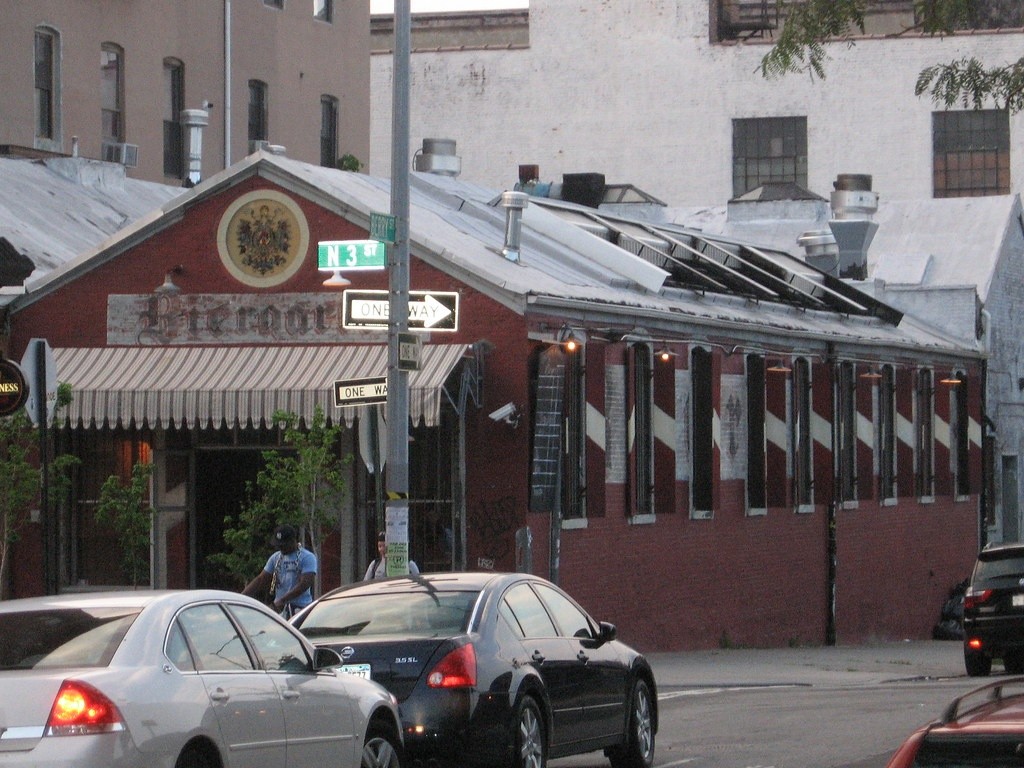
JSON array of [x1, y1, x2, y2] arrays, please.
[[0, 582, 408, 768], [286, 568, 661, 767]]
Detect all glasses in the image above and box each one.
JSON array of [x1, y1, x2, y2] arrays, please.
[[378, 531, 385, 537]]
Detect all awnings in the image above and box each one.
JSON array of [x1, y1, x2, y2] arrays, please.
[[33, 344, 466, 429]]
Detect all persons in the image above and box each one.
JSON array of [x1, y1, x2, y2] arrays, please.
[[419, 505, 449, 557], [363, 532, 421, 580], [241, 524, 318, 622]]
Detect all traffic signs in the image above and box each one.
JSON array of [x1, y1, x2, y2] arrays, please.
[[339, 286, 460, 335], [330, 376, 388, 409], [395, 330, 423, 372]]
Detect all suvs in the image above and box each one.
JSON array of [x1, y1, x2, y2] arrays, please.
[[961, 539, 1024, 678], [882, 675, 1024, 768]]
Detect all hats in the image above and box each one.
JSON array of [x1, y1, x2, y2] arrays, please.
[[273, 524, 294, 546]]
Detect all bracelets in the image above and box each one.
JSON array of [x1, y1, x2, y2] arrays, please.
[[272, 602, 278, 609]]
